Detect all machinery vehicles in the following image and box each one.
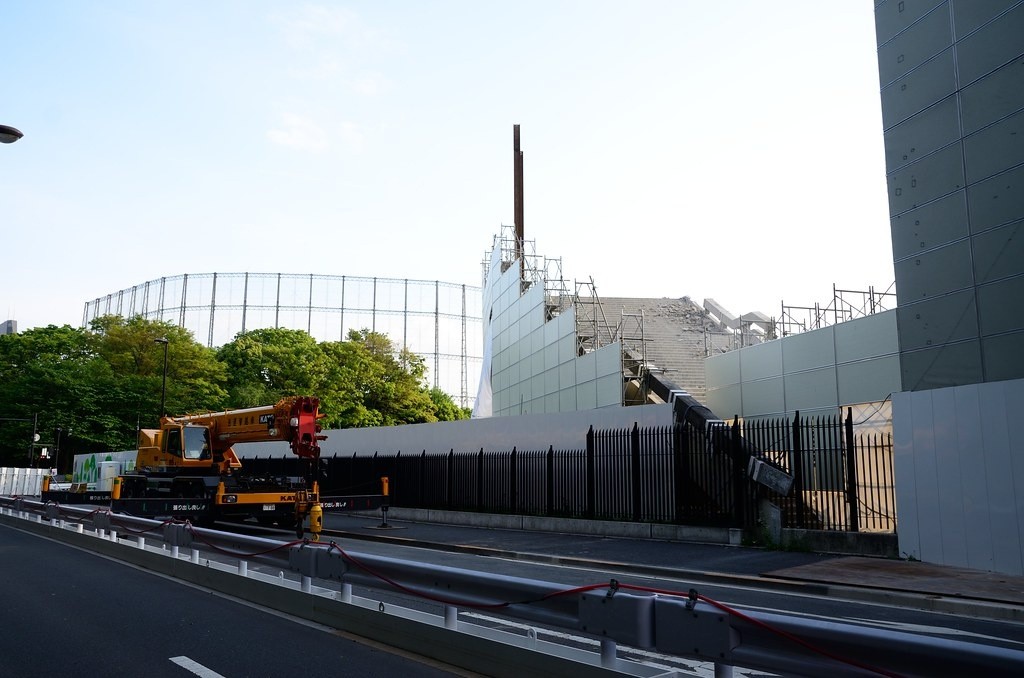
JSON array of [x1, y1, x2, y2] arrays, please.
[[108, 395, 391, 541]]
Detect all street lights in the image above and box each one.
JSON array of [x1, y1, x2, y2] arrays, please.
[[154, 340, 169, 417], [55, 425, 62, 468]]
